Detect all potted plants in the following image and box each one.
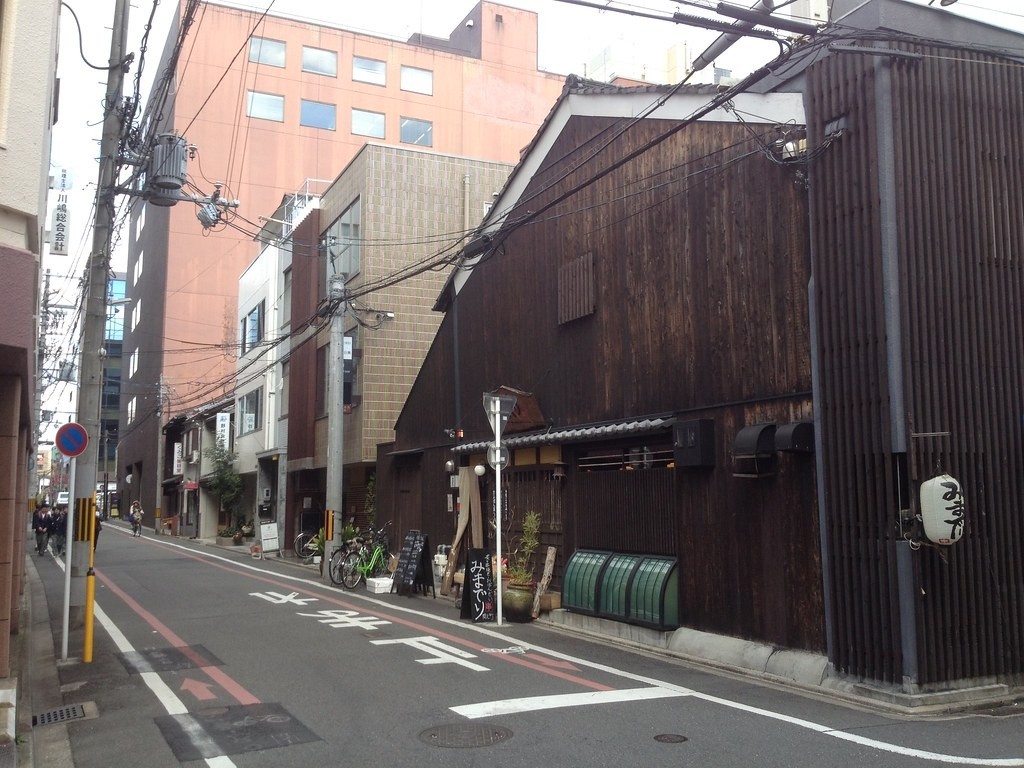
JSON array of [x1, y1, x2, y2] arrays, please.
[[503, 508, 545, 623]]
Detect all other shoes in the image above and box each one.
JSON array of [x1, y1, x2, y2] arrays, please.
[[44, 550, 47, 552]]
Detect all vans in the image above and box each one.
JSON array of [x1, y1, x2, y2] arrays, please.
[[95, 491, 117, 510]]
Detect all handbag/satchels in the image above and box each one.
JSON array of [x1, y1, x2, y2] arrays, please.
[[128, 513, 134, 522]]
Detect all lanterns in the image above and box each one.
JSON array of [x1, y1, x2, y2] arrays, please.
[[920, 474, 965, 545]]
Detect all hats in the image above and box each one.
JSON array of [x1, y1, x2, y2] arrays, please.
[[133, 500, 139, 505]]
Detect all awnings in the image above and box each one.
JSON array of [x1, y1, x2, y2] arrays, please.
[[178, 481, 199, 489], [161, 476, 183, 487]]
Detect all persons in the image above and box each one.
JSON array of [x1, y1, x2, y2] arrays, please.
[[32, 503, 68, 557], [129, 500, 144, 532], [94, 505, 102, 551]]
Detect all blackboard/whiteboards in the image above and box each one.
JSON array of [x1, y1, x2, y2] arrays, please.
[[393, 533, 425, 586], [469, 551, 495, 623]]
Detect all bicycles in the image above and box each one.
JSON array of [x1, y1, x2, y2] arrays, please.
[[131, 513, 144, 537], [293, 527, 320, 559], [329, 520, 396, 592]]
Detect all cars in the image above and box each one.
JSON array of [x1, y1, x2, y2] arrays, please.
[[53, 491, 69, 508]]
